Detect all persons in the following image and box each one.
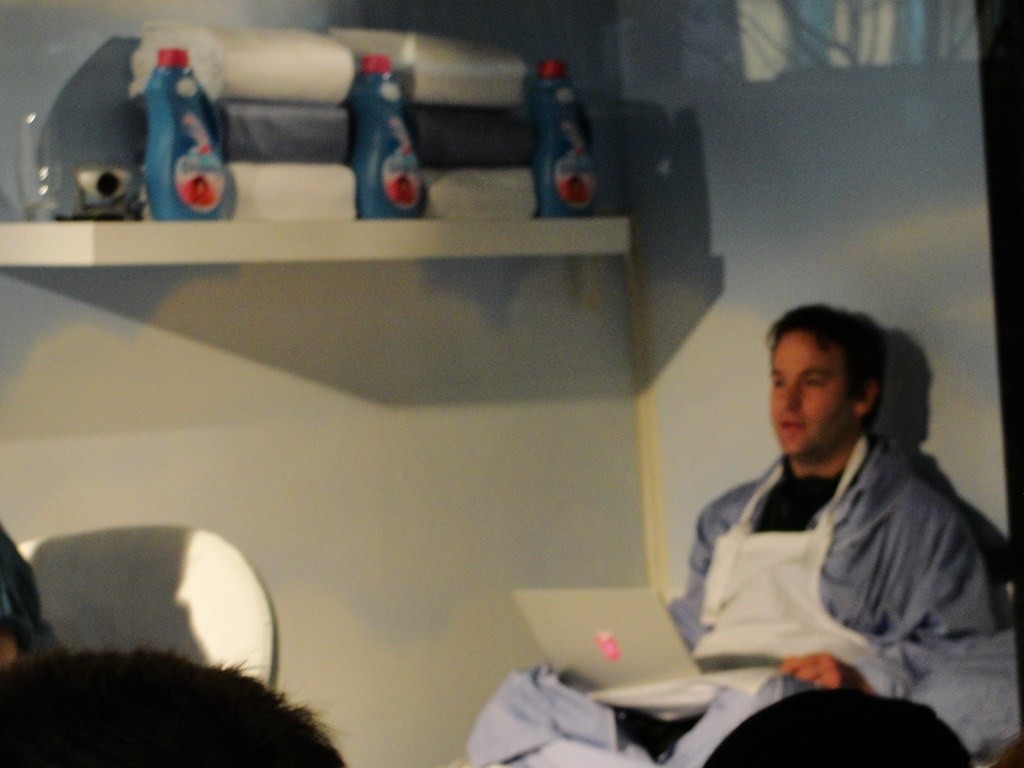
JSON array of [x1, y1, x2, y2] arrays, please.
[[554, 302, 994, 768]]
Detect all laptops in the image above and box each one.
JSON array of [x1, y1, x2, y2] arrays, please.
[[511, 588, 700, 695]]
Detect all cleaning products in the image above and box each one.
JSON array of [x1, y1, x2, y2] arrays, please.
[[350, 54, 428, 219], [528, 59, 599, 217], [142, 47, 237, 221]]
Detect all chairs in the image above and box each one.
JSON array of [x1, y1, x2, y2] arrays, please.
[[11, 523, 276, 689]]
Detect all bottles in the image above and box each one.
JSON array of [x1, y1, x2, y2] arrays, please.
[[530, 61, 604, 220], [349, 57, 423, 220], [144, 48, 236, 219]]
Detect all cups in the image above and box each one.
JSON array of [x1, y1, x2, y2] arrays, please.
[[16, 106, 64, 218]]
